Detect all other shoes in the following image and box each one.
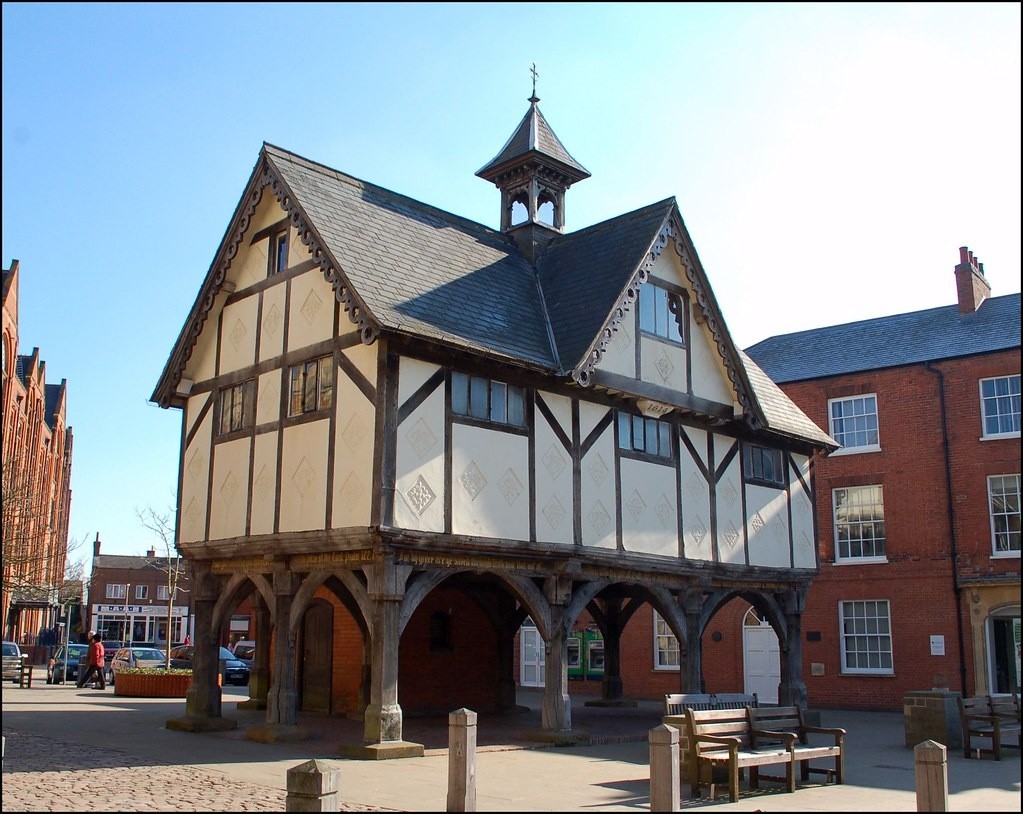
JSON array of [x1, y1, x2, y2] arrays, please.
[[98, 687, 105, 690], [75, 683, 82, 688], [92, 687, 100, 689]]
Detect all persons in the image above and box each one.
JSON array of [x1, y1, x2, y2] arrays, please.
[[227, 641, 235, 653], [77, 630, 106, 690], [136, 626, 142, 641], [184, 635, 190, 645]]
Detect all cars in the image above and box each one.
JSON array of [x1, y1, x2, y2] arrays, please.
[[47, 644, 91, 685], [109, 647, 172, 686], [169, 644, 249, 686], [100, 641, 122, 661]]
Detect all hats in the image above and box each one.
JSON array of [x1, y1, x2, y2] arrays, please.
[[92, 634, 101, 642]]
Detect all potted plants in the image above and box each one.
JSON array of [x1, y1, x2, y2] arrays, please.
[[114, 667, 193, 697]]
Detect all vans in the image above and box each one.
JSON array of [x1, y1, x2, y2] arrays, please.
[[1, 641, 29, 683], [232, 640, 256, 670]]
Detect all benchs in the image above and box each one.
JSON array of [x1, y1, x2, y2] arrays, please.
[[684, 704, 847, 803], [957, 693, 1021, 761]]
